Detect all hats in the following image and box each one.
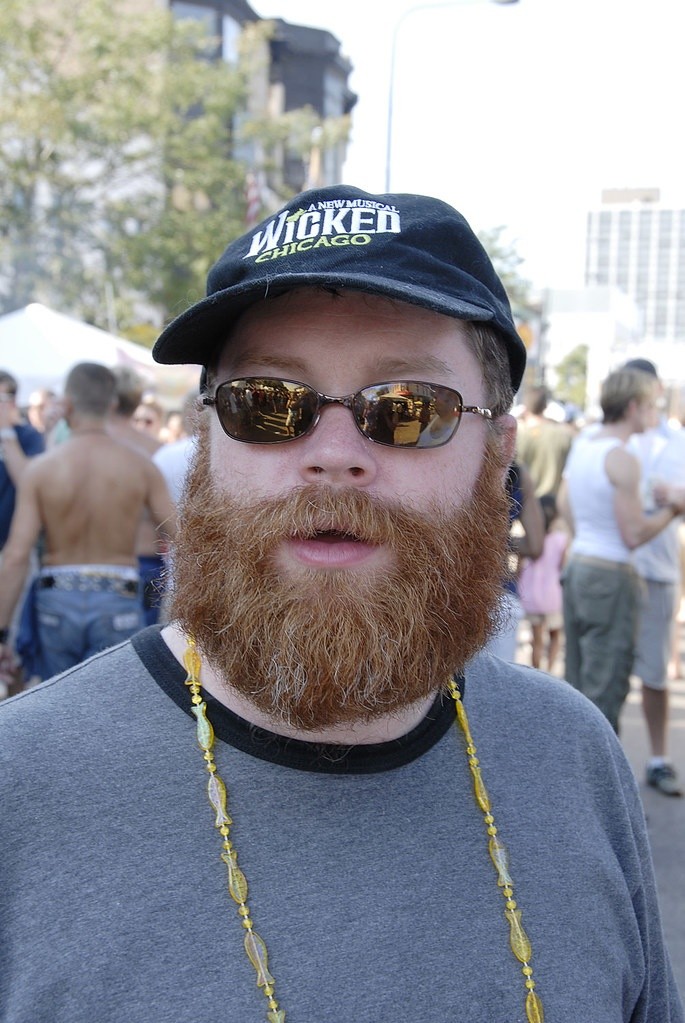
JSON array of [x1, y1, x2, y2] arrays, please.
[[153, 185, 526, 397]]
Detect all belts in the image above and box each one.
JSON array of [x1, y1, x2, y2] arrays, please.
[[39, 573, 138, 594]]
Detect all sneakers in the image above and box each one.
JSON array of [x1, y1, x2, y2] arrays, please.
[[646, 763, 682, 794]]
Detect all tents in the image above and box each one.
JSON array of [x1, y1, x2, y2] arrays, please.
[[2, 301, 192, 384]]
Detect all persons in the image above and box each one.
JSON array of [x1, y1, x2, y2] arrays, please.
[[0, 183, 685, 1023], [0, 356, 685, 796]]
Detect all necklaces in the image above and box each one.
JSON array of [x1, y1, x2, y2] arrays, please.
[[182, 630, 548, 1023]]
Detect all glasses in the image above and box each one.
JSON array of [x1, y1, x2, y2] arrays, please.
[[134, 418, 156, 425], [201, 377, 498, 449]]
